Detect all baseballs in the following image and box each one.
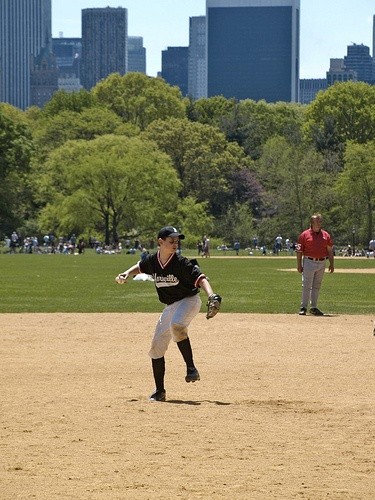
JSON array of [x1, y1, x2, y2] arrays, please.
[[116, 276, 124, 284]]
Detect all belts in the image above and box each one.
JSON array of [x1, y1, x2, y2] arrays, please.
[[308, 256, 328, 261]]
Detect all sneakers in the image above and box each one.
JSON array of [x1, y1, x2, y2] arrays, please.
[[309, 308, 324, 316], [299, 307, 307, 315], [151, 389, 166, 400], [185, 368, 200, 383]]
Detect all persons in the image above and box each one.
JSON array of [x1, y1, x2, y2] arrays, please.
[[115, 227, 221, 401], [296, 213, 334, 316], [0, 232, 375, 259]]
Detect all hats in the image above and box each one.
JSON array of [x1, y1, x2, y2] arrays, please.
[[158, 226, 185, 240]]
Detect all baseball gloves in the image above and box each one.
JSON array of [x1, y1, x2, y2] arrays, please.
[[205, 293, 222, 319]]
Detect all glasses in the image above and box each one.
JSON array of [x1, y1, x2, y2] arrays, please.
[[165, 238, 178, 244]]
